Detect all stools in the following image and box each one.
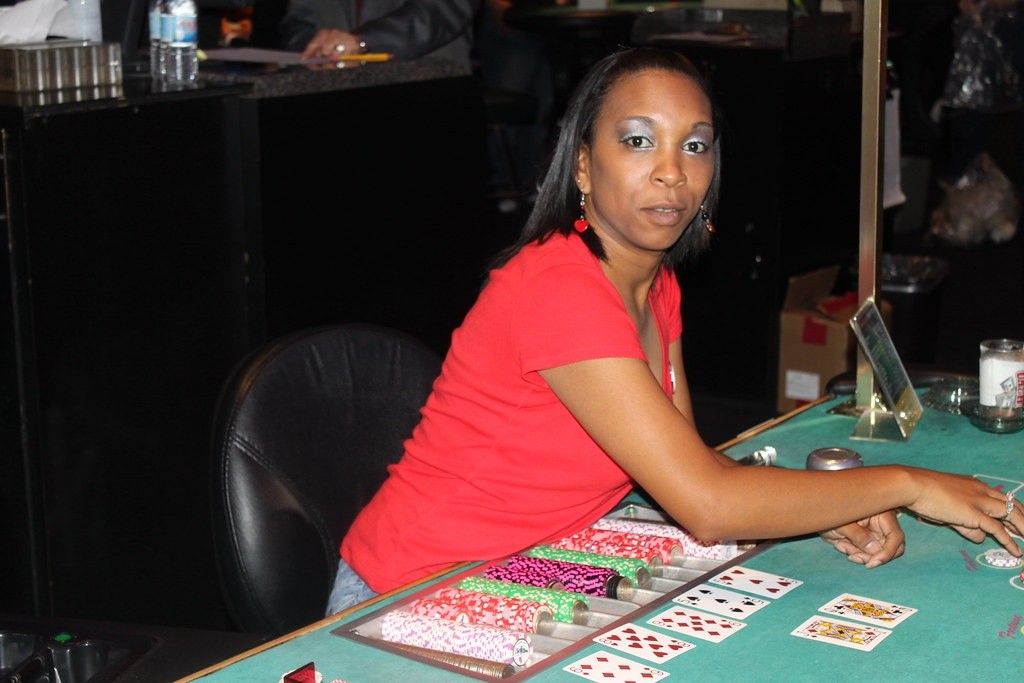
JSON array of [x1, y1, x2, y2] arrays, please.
[[468, 5, 642, 198]]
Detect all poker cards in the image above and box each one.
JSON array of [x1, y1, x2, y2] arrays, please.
[[561, 563, 922, 683]]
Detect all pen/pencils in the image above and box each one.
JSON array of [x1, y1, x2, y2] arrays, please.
[[327, 53, 396, 60]]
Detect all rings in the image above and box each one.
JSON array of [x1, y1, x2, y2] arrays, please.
[[335, 62, 346, 70], [334, 43, 346, 53], [1000, 491, 1015, 520]]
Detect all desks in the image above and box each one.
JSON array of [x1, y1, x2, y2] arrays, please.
[[0, 39, 473, 370], [173, 364, 1024, 683]]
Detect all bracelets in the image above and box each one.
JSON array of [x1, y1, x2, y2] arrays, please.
[[350, 31, 369, 50]]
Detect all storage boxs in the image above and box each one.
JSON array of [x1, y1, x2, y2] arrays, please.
[[776, 266, 894, 416]]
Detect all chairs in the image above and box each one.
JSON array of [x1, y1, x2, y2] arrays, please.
[[206, 319, 443, 635]]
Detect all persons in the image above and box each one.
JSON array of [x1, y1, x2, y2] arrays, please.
[[304, 60, 367, 72], [324, 48, 1024, 616], [277, 0, 472, 60]]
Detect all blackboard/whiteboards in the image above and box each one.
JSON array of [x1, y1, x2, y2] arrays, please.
[[850, 297, 910, 408]]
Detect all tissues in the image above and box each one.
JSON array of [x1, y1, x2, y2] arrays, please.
[[0, 0, 125, 109]]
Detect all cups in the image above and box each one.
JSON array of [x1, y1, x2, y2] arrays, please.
[[0, 633, 35, 677], [49, 638, 108, 683], [977, 339, 1024, 419]]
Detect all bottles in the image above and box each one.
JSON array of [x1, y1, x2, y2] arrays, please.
[[149, 0, 198, 84]]
